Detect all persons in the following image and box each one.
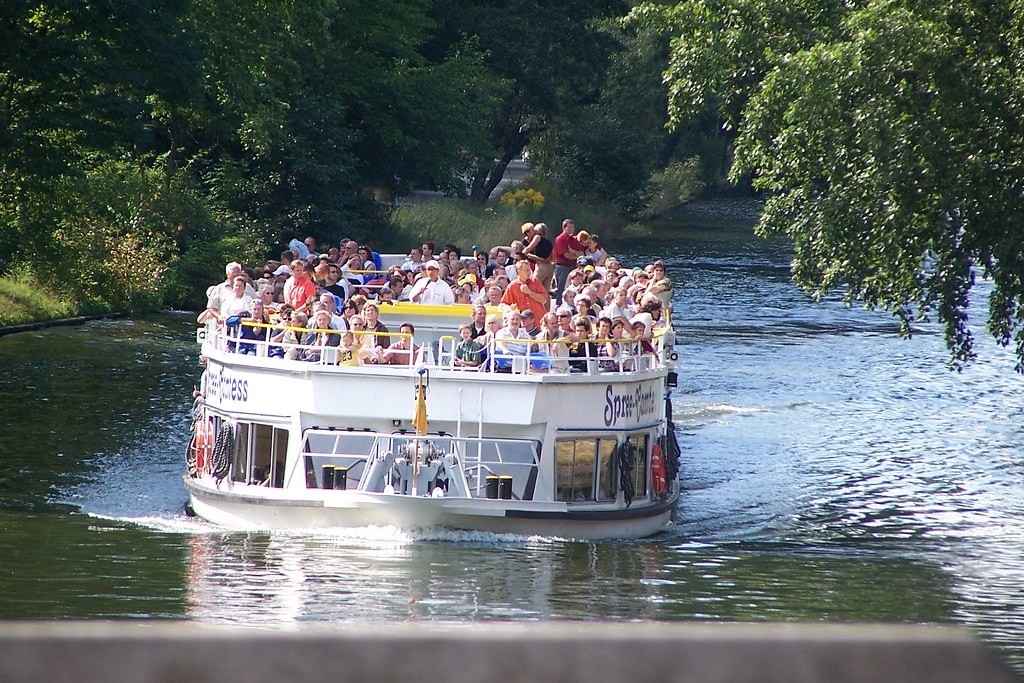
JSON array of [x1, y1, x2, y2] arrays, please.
[[197, 218, 673, 373], [259, 460, 284, 488]]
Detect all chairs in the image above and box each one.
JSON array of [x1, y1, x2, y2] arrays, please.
[[484, 350, 515, 374], [530, 353, 551, 373], [371, 252, 383, 279], [334, 295, 342, 315]]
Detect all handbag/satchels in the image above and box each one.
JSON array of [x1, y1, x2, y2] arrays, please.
[[641, 299, 662, 321]]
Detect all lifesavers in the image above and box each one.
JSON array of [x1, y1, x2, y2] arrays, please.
[[192, 419, 208, 475], [650, 444, 668, 497]]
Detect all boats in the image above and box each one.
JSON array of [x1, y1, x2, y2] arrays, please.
[[181, 254, 681, 542]]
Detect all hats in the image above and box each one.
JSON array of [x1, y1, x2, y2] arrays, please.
[[272, 265, 290, 275], [580, 258, 594, 266], [426, 260, 440, 270], [519, 309, 534, 318], [632, 267, 641, 276], [584, 265, 595, 274], [466, 274, 476, 284], [252, 299, 263, 306], [296, 311, 308, 325], [457, 279, 467, 286]]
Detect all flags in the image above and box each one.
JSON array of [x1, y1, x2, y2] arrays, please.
[[411, 371, 428, 436]]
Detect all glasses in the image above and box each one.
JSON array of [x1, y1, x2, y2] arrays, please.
[[283, 318, 292, 321], [329, 271, 338, 274], [277, 273, 285, 276], [350, 324, 362, 325], [263, 292, 274, 295], [478, 258, 486, 260], [557, 314, 569, 318], [264, 277, 272, 279], [344, 306, 354, 310], [654, 270, 664, 272]]
